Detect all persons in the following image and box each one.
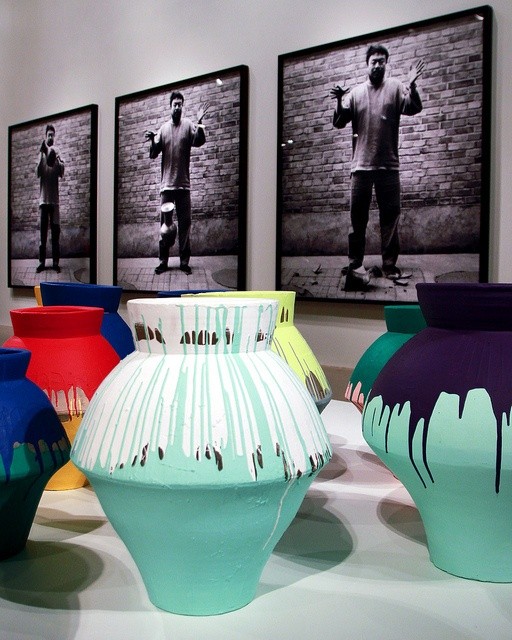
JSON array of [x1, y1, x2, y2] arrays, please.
[[35, 124, 65, 273], [329, 45, 426, 276], [142, 90, 210, 275]]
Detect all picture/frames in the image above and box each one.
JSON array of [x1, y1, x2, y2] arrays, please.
[[111, 64, 247, 296], [7, 104, 99, 290], [275, 4, 492, 305]]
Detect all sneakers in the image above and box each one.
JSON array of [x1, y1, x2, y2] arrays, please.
[[382, 264, 399, 278], [155, 262, 168, 274], [179, 264, 192, 273], [341, 264, 355, 275], [52, 264, 61, 273], [36, 263, 45, 272]]
